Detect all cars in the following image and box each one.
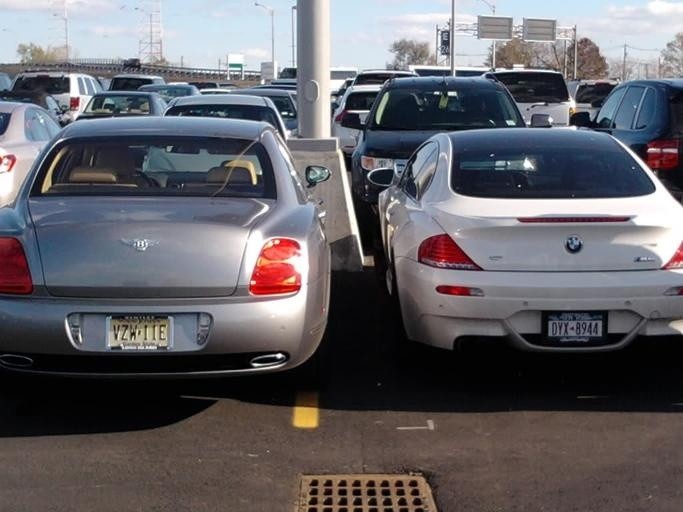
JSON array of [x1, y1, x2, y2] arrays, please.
[[123, 58, 142, 69], [357, 117, 683, 368], [1, 108, 351, 394]]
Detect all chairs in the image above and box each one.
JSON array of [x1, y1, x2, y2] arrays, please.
[[95, 142, 147, 185], [188, 165, 255, 190], [220, 159, 258, 185], [56, 164, 132, 187]]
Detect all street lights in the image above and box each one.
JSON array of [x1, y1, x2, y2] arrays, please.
[[133, 7, 154, 62], [51, 11, 69, 62], [291, 5, 297, 66], [254, 1, 276, 79]]
